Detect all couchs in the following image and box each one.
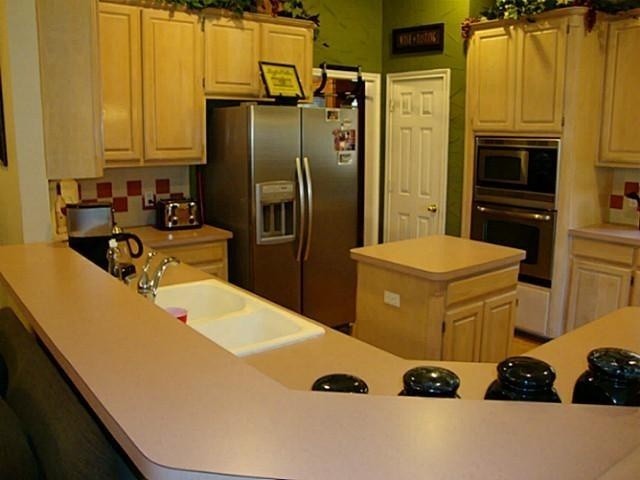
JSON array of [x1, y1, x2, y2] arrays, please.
[[1, 306, 137, 479]]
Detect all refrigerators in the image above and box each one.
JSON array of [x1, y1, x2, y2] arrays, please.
[[195, 105, 358, 327]]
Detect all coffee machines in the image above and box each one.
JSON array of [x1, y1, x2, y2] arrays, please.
[[68, 202, 143, 281]]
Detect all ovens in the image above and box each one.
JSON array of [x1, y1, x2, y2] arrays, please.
[[470, 137, 561, 287]]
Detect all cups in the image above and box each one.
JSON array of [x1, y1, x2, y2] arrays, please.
[[164, 308, 188, 324]]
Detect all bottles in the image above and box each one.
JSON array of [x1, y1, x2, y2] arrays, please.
[[481, 358, 563, 405], [566, 347, 640, 407], [310, 373, 371, 396], [397, 365, 463, 399]]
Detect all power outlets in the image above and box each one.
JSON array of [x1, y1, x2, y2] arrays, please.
[[145, 191, 154, 209]]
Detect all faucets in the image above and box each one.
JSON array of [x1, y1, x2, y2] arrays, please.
[[137, 249, 180, 296]]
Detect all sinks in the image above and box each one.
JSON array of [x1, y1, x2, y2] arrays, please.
[[156, 278, 245, 320], [192, 308, 325, 358]]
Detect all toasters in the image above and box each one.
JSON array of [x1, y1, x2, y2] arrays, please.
[[154, 197, 202, 231]]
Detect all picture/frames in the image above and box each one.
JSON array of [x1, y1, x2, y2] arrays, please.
[[391, 22, 443, 55], [258, 61, 307, 100]]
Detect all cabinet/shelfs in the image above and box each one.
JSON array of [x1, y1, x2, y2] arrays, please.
[[98, 0, 206, 169], [465, 9, 610, 139], [563, 237, 640, 335], [201, 6, 315, 103], [348, 235, 526, 363], [36, 1, 104, 180], [158, 242, 228, 282], [595, 10, 640, 170]]
